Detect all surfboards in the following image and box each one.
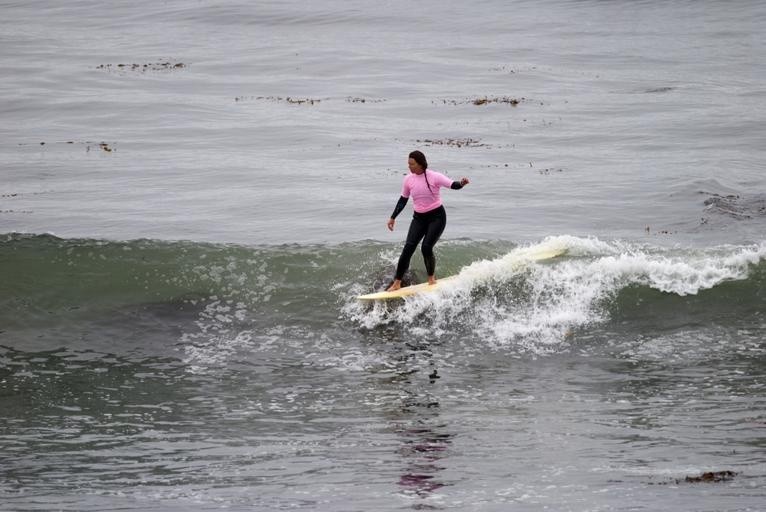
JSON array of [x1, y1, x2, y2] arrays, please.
[[357, 276, 452, 301]]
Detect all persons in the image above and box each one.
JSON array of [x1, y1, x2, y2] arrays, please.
[[387, 150, 470, 291]]
